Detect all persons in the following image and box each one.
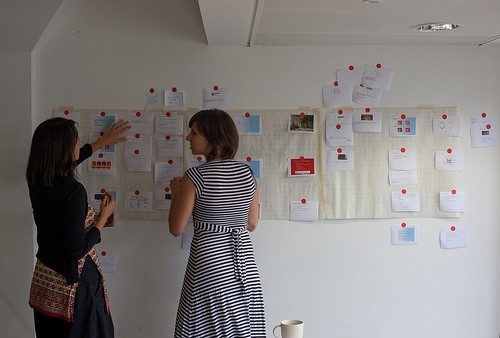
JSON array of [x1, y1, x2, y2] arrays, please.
[[165, 108, 267, 338], [26, 116, 132, 338]]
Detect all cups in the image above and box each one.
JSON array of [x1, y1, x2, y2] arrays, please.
[[273, 319, 304, 338]]
